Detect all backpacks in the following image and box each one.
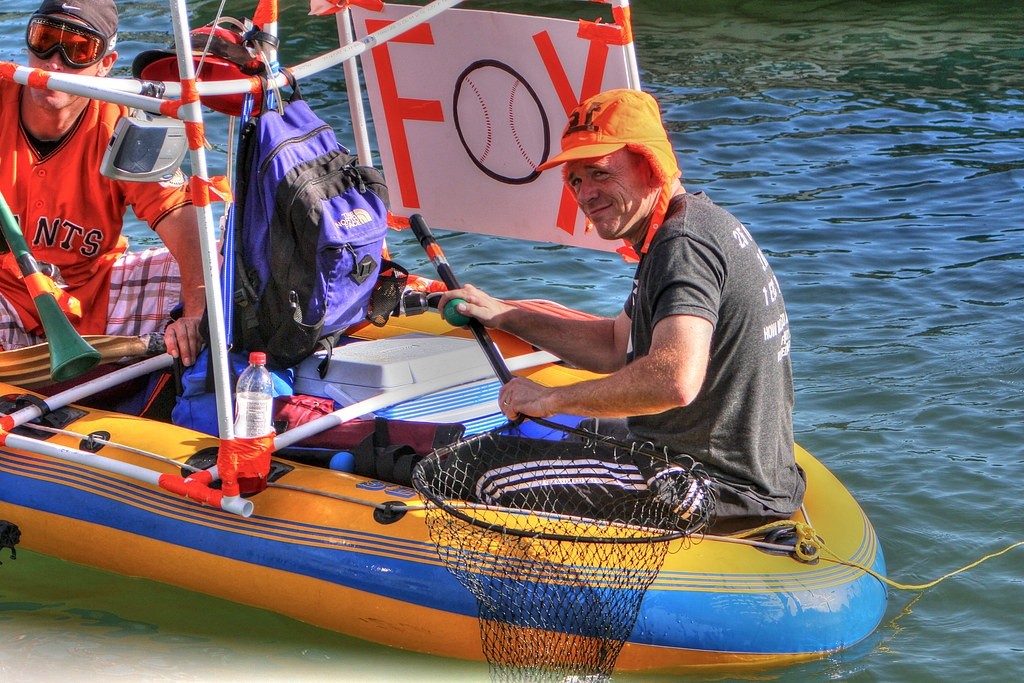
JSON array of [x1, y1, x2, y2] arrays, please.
[[219, 62, 409, 364]]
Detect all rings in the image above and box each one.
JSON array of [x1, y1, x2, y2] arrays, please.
[[504, 400, 509, 407]]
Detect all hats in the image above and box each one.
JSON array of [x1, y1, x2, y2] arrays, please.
[[39, 0, 120, 51], [535, 82, 681, 224], [129, 11, 267, 116]]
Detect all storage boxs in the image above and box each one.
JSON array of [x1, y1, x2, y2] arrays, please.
[[297, 334, 519, 438]]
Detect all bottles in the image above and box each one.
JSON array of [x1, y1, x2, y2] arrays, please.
[[233, 351, 275, 438]]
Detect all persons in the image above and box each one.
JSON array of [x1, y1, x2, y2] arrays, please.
[[437, 88, 808, 536], [0, 1, 207, 367]]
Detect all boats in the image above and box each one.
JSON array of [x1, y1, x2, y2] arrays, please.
[[0, 0, 900, 676]]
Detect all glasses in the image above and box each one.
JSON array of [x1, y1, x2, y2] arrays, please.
[[25, 17, 110, 69]]
